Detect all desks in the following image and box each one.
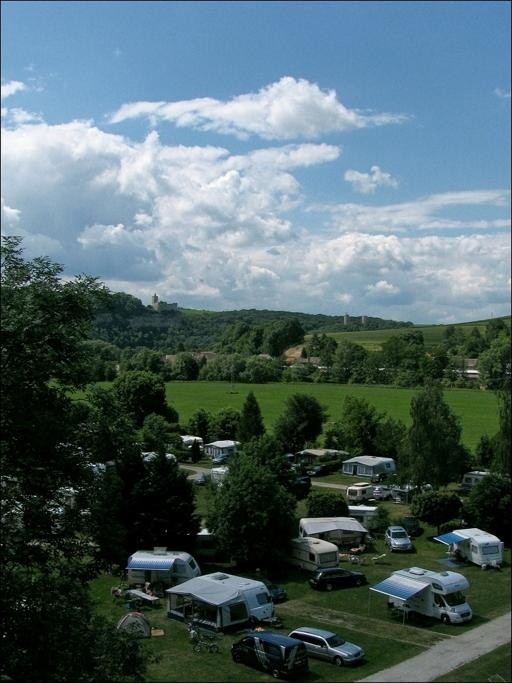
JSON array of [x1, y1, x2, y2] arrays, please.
[[127, 589, 159, 607]]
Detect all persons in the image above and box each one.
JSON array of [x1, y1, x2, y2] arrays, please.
[[142, 582, 153, 596], [190, 628, 198, 651]]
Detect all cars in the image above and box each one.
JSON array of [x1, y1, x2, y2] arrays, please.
[[385, 515, 420, 552], [289, 627, 365, 666], [307, 465, 328, 476], [249, 574, 287, 600], [213, 454, 228, 465], [371, 472, 390, 482], [308, 568, 367, 591], [195, 478, 205, 485]]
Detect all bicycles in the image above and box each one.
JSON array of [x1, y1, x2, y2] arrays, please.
[[193, 638, 219, 654]]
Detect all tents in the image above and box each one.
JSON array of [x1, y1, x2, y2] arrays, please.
[[117, 611, 153, 640]]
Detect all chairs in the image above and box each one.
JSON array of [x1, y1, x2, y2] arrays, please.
[[387, 602, 399, 619]]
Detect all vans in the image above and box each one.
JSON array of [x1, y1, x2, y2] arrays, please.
[[231, 632, 308, 678], [370, 567, 473, 626], [347, 482, 373, 502]]
